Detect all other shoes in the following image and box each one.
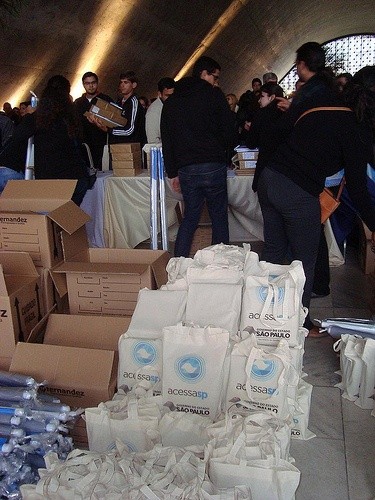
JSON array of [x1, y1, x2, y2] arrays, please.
[[310, 286, 330, 295], [307, 326, 328, 338]]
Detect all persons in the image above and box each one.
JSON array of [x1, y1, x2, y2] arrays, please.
[[0, 71, 175, 171], [160, 55, 240, 258], [33, 74, 88, 208], [226, 42, 375, 338]]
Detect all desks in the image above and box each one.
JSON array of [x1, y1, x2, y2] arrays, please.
[[103, 170, 177, 250], [228, 171, 264, 244]]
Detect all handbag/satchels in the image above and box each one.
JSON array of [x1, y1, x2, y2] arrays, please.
[[82, 142, 97, 176], [319, 187, 341, 223], [333, 334, 375, 417], [20, 243, 316, 500]]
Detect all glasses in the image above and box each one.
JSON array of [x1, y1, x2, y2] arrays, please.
[[210, 73, 219, 81]]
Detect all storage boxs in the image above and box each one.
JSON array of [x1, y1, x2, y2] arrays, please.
[[233, 148, 259, 168], [0, 100, 169, 448], [358, 219, 375, 273]]
